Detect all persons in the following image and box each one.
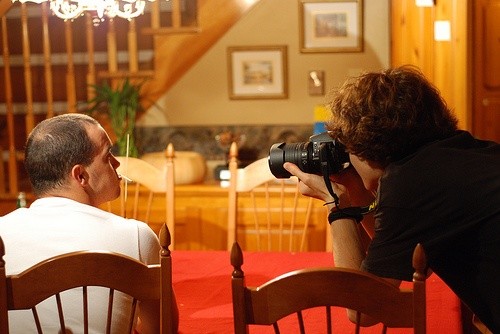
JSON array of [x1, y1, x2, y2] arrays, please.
[[0, 113, 177, 334], [282, 65, 500, 334]]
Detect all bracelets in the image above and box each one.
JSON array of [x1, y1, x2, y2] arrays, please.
[[328, 208, 365, 223]]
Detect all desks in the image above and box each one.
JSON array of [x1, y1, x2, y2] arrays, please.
[[170, 249, 464, 334]]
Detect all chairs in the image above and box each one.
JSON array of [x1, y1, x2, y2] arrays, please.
[[108, 142, 176, 251], [230, 241, 427, 334], [0, 222, 172, 334], [226, 141, 334, 253]]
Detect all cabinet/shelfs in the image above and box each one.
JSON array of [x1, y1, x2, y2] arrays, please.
[[99, 179, 334, 252]]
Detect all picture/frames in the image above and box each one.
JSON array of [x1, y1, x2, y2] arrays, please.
[[297, 0, 364, 54], [226, 44, 289, 100]]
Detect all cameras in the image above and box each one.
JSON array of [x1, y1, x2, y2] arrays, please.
[[268, 132, 353, 179]]
